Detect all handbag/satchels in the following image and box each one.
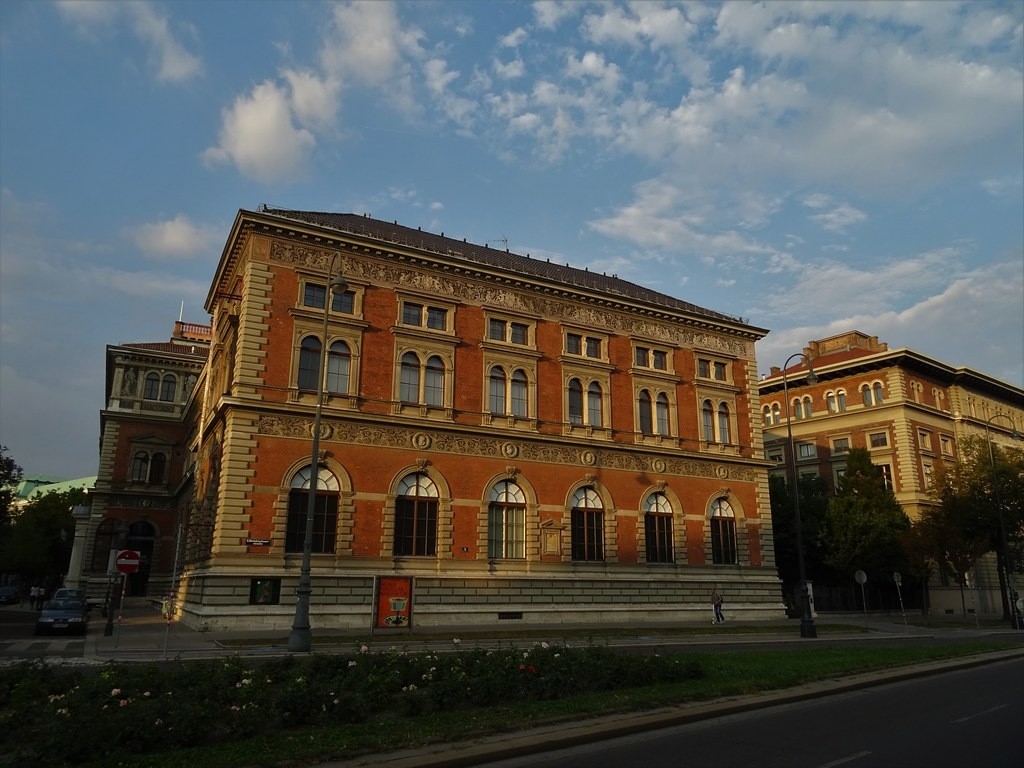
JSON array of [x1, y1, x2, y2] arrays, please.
[[718, 596, 723, 603]]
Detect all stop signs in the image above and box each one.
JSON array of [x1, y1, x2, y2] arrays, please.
[[115, 552, 140, 573]]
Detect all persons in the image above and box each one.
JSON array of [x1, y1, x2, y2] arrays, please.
[[29, 584, 45, 611], [710, 588, 725, 624]]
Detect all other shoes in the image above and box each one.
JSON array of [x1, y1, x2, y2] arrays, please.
[[30, 606, 33, 610], [722, 620, 726, 624], [35, 606, 42, 611], [716, 621, 720, 624]]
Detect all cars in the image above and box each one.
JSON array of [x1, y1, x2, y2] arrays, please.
[[35, 597, 89, 636], [52, 587, 90, 613], [0, 586, 20, 605]]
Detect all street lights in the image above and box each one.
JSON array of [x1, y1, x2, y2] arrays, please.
[[986, 415, 1024, 631], [290, 250, 350, 653], [783, 353, 820, 638]]
